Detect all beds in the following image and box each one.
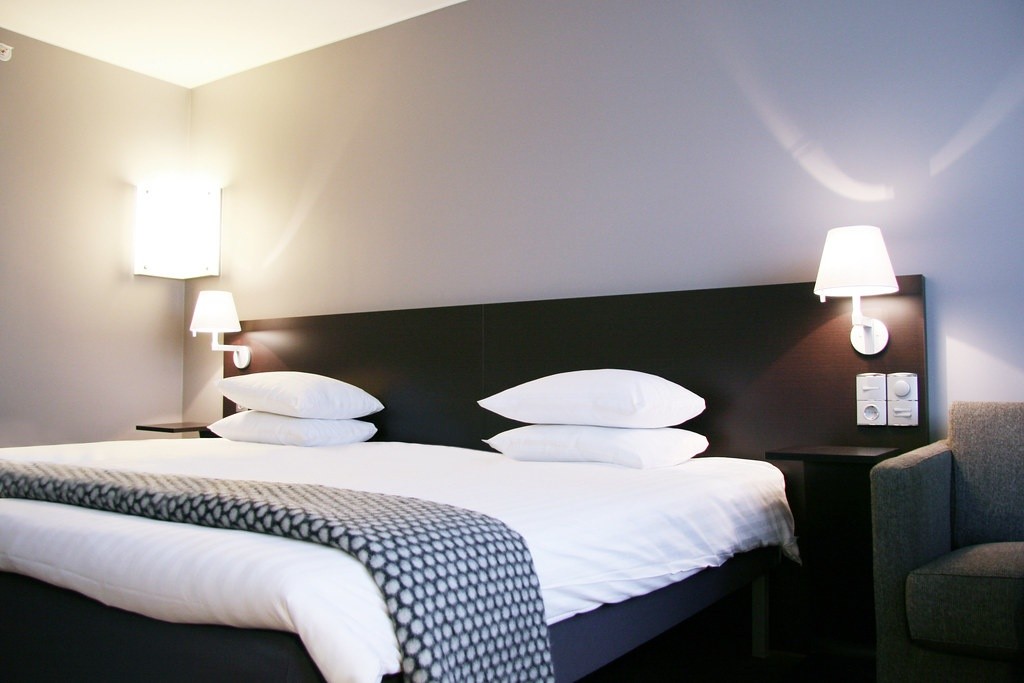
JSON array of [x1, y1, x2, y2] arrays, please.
[[0, 271, 934, 682]]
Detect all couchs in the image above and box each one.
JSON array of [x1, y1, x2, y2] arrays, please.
[[870, 398, 1023, 675]]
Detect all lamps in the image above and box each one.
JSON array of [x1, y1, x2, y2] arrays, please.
[[812, 223, 899, 356], [189, 290, 253, 370]]
